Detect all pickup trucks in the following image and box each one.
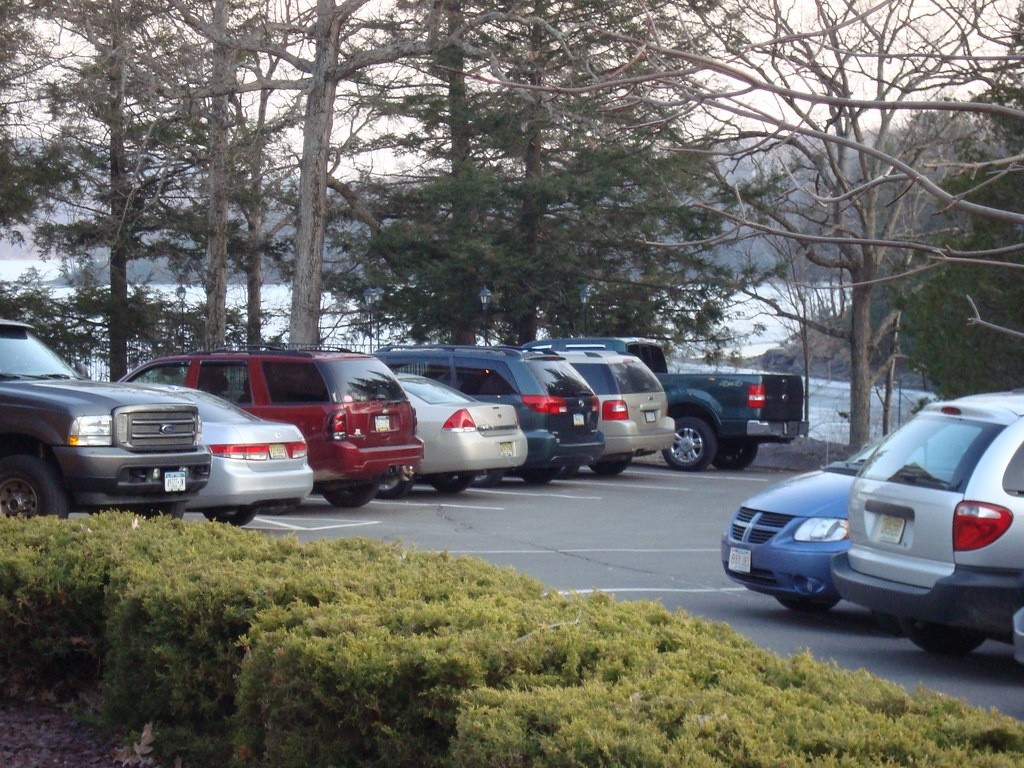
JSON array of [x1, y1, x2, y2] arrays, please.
[[516, 333, 812, 475]]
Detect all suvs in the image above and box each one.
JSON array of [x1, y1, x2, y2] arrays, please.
[[0, 317, 213, 527], [522, 339, 679, 476], [369, 342, 607, 490], [113, 343, 426, 509]]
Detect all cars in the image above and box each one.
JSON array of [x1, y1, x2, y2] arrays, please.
[[125, 380, 314, 529], [718, 433, 905, 614], [830, 388, 1024, 657], [374, 371, 528, 500]]
